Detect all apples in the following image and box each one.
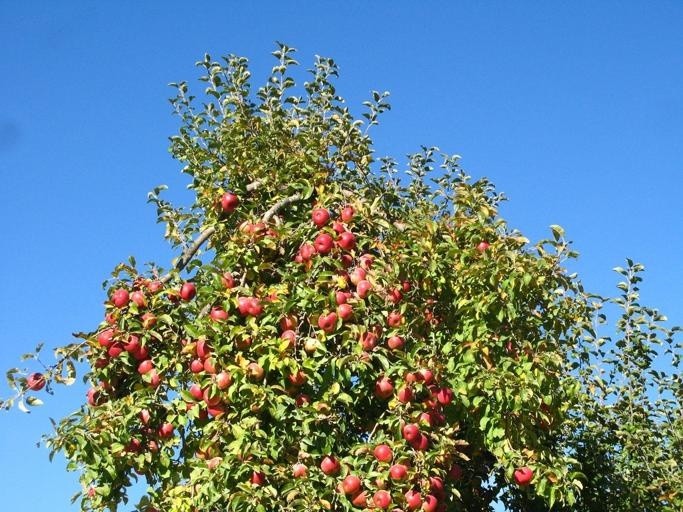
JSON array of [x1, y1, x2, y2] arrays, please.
[[26, 191, 534, 512]]
